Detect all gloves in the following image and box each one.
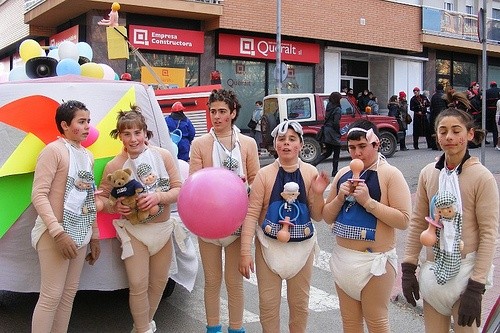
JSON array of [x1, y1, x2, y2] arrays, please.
[[457, 277, 485, 327], [401, 263, 420, 306]]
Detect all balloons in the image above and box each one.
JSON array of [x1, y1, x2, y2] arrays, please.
[[177, 167, 248, 239], [7, 39, 120, 80]]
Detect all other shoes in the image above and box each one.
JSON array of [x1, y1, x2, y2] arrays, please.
[[494, 146, 500, 151], [485, 140, 490, 144], [413, 145, 419, 149], [400, 146, 408, 151]]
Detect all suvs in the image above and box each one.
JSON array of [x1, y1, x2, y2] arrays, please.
[[259, 93, 405, 166]]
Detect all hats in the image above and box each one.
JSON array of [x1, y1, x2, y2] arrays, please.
[[348, 88, 353, 91], [490, 81, 497, 85], [413, 87, 420, 91], [399, 92, 405, 100], [471, 82, 478, 86]]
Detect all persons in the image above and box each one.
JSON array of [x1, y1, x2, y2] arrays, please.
[[347, 88, 381, 114], [121, 72, 131, 82], [190, 90, 261, 333], [248, 101, 263, 155], [96, 2, 120, 26], [403, 108, 499, 333], [312, 92, 342, 177], [239, 120, 330, 333], [165, 102, 196, 160], [322, 119, 412, 333], [31, 99, 104, 333], [387, 81, 500, 149], [98, 105, 184, 333]]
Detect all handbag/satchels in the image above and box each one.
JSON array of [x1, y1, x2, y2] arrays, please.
[[315, 126, 324, 141], [248, 119, 257, 129], [406, 114, 412, 124]]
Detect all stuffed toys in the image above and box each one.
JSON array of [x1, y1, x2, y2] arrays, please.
[[107, 168, 150, 225]]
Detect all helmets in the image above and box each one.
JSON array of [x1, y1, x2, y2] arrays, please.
[[170, 101, 186, 112]]
